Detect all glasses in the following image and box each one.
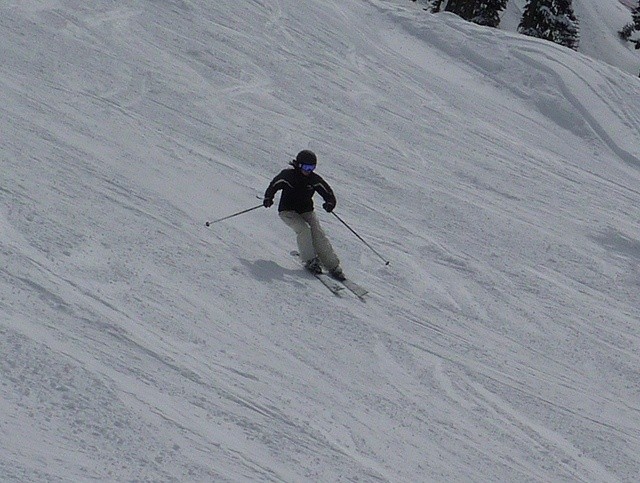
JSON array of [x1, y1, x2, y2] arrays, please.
[[299, 163, 316, 171]]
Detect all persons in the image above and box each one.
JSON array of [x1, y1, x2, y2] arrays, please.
[[263, 150, 347, 281]]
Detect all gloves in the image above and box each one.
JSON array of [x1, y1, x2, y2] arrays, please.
[[264, 198, 273, 208], [323, 200, 335, 212]]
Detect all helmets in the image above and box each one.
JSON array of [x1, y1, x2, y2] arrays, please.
[[297, 150, 316, 165]]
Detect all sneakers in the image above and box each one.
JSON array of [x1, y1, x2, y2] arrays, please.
[[308, 262, 321, 274], [329, 267, 345, 281]]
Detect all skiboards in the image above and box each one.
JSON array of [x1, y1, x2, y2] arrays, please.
[[289, 251, 369, 298]]
